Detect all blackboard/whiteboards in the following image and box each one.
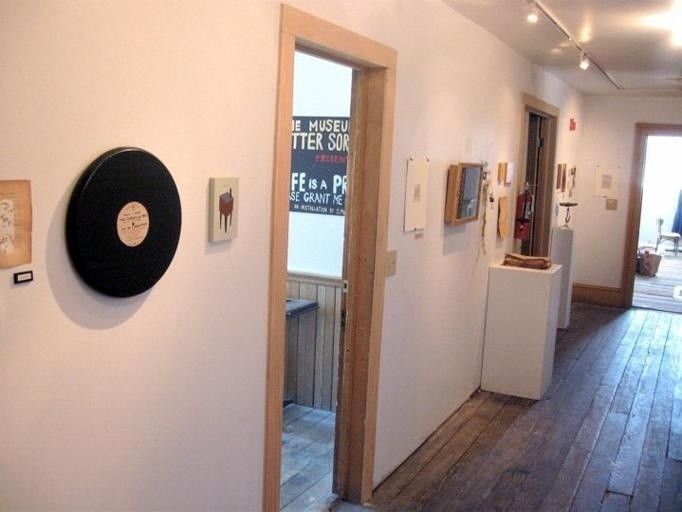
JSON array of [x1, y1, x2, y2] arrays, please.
[[289, 117, 350, 215]]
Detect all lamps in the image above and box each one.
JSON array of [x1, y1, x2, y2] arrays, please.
[[527, 1, 591, 70]]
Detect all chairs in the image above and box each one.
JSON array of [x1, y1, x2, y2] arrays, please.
[[655, 215, 681, 257]]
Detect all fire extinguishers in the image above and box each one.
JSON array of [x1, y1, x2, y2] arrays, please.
[[514, 182, 532, 241]]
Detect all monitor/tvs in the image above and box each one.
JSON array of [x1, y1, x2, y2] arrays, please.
[[445, 163, 484, 226]]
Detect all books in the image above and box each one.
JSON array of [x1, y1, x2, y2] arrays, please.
[[503, 252, 552, 270]]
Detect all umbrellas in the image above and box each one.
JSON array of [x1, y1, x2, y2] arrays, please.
[[670, 190, 682, 239]]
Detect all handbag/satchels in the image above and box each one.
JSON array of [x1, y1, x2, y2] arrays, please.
[[635, 250, 662, 276]]
